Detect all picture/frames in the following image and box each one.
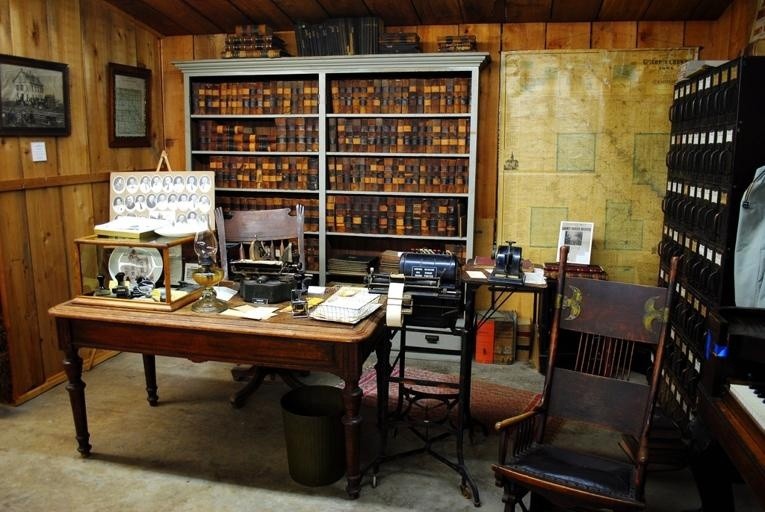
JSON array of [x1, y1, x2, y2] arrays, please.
[[106, 62, 152, 148], [556, 221, 594, 265], [0, 54, 70, 137]]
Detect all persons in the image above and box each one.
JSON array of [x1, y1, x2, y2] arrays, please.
[[114, 175, 211, 224]]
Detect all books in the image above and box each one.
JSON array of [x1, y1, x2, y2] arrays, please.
[[191, 81, 319, 115], [307, 283, 382, 326], [191, 117, 318, 152], [464, 254, 534, 273], [327, 249, 409, 274], [328, 157, 468, 193], [473, 309, 533, 366], [192, 154, 319, 191], [331, 79, 470, 114], [221, 24, 290, 58], [94, 215, 165, 239], [328, 118, 470, 154], [294, 14, 423, 57], [437, 35, 478, 52], [215, 195, 319, 271], [326, 195, 467, 237]]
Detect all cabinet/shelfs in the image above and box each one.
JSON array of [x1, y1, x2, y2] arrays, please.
[[641, 57, 765, 467], [170, 51, 489, 363]]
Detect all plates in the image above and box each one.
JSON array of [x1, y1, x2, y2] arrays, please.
[[108, 247, 163, 290]]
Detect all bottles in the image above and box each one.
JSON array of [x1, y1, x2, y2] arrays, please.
[[291, 273, 309, 319], [93, 272, 145, 299]]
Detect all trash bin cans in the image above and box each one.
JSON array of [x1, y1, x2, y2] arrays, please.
[[280, 385, 344, 486]]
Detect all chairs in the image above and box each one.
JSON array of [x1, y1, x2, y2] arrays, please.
[[491, 246, 680, 512], [213, 203, 310, 409]]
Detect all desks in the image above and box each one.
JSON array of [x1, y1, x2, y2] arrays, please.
[[462, 258, 548, 360], [542, 262, 609, 357], [48, 280, 393, 500]]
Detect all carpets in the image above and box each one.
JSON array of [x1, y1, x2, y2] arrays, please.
[[336, 363, 543, 427]]
